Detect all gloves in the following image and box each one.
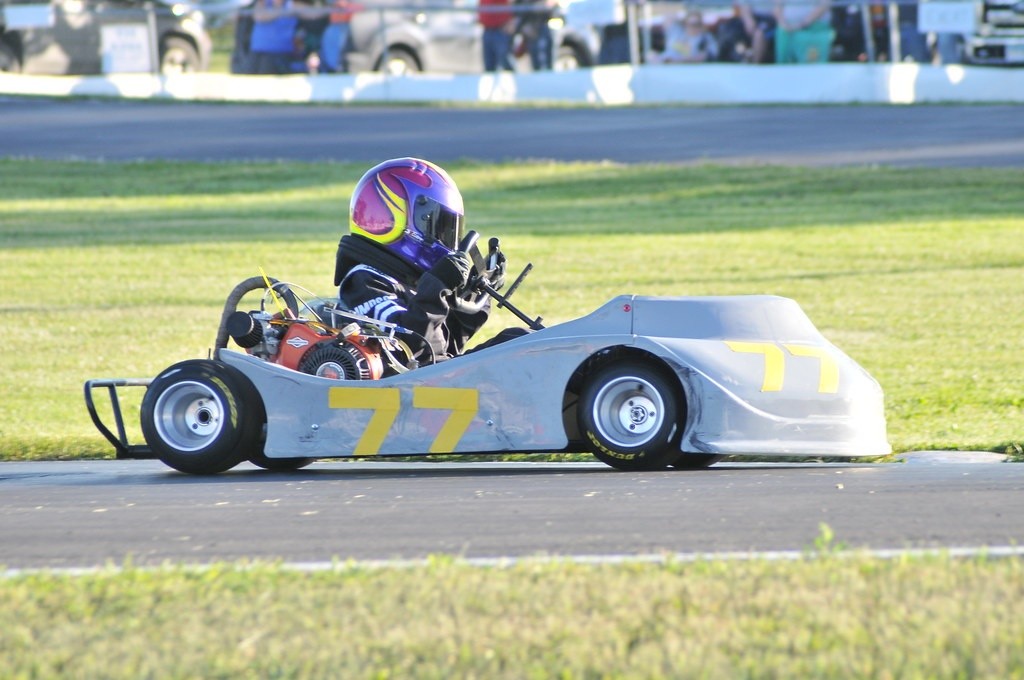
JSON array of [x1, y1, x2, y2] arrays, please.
[[469, 249, 507, 292], [447, 250, 477, 292]]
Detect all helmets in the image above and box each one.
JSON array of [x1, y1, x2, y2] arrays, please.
[[335, 157, 464, 287]]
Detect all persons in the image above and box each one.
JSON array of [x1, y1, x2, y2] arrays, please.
[[653, 1, 839, 62], [246, 0, 358, 75], [476, 1, 558, 72], [332, 156, 534, 368]]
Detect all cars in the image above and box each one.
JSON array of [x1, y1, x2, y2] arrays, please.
[[347, 0, 598, 76], [1, 0, 217, 75]]
